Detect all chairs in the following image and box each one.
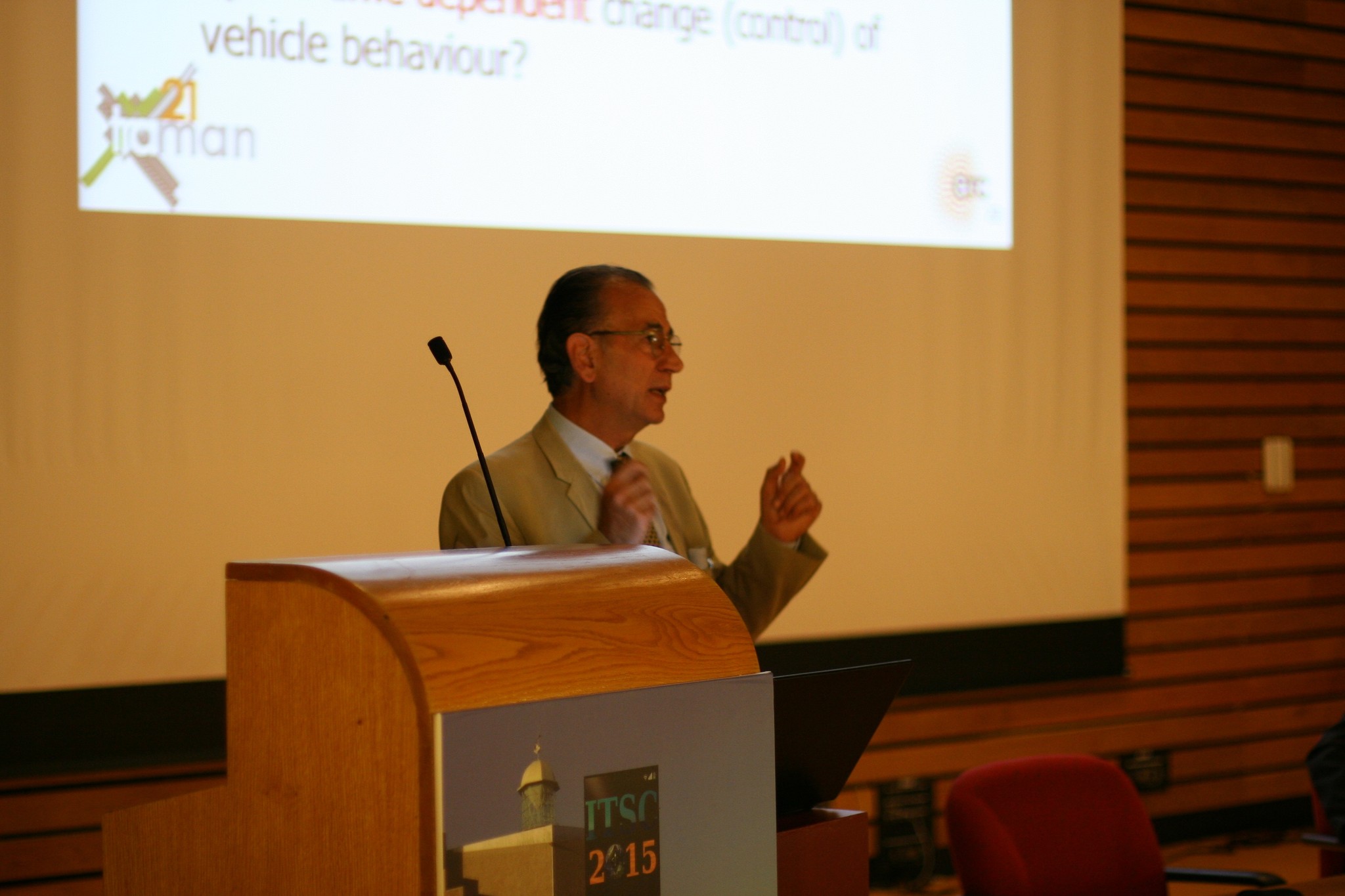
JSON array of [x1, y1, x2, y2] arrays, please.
[[943, 749, 1289, 896], [1298, 773, 1344, 881]]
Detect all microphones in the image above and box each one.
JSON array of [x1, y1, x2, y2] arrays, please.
[[426, 335, 513, 546]]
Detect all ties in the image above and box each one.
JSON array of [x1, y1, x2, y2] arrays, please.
[[608, 456, 661, 549]]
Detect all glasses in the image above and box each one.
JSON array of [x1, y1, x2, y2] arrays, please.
[[588, 328, 682, 358]]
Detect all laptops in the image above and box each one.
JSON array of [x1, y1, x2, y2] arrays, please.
[[772, 655, 913, 820]]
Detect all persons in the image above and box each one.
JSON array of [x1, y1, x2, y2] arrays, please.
[[436, 264, 828, 645]]
